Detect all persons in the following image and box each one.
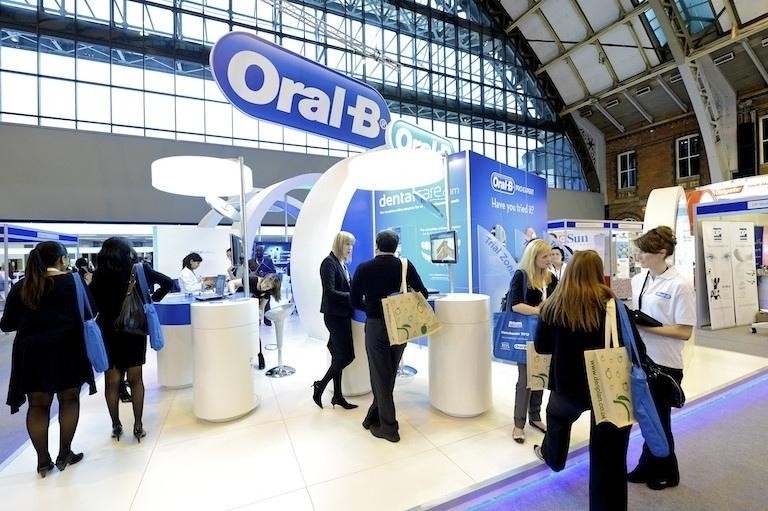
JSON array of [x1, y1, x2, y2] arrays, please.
[[177, 251, 217, 295], [75, 257, 94, 286], [85, 236, 174, 445], [349, 230, 430, 445], [308, 229, 359, 412], [627, 224, 697, 491], [227, 272, 283, 371], [0, 240, 97, 479], [226, 247, 252, 279], [247, 244, 278, 328], [510, 238, 648, 510]]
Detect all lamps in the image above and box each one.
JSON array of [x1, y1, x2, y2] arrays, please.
[[152, 157, 253, 197]]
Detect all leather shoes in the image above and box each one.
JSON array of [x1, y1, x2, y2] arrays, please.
[[370, 425, 399, 441], [529, 420, 547, 434], [534, 445, 543, 460], [363, 419, 372, 429], [513, 427, 525, 443]]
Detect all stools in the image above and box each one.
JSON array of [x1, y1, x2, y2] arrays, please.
[[265, 303, 294, 377]]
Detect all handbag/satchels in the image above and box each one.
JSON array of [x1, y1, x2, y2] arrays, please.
[[641, 362, 685, 408], [81, 266, 93, 285], [616, 299, 669, 457], [526, 341, 552, 390], [72, 272, 108, 372], [383, 258, 441, 346], [135, 262, 164, 350], [584, 297, 637, 428], [492, 268, 539, 363]]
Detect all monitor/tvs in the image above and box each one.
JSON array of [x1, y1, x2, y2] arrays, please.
[[231, 233, 241, 267], [430, 231, 457, 264]]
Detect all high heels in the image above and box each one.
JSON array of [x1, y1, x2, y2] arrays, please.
[[134, 427, 146, 443], [332, 396, 358, 409], [311, 380, 323, 408], [56, 453, 83, 471], [112, 425, 122, 441], [38, 461, 54, 477]]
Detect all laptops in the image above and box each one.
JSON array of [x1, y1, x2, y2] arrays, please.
[[194, 274, 226, 301]]
